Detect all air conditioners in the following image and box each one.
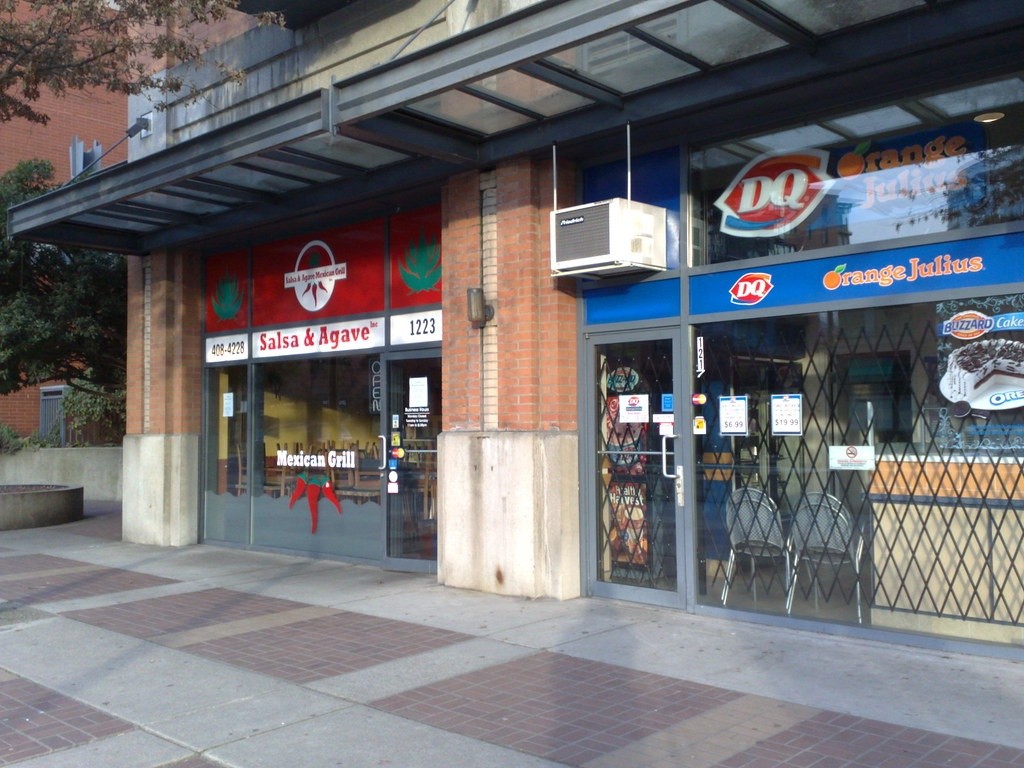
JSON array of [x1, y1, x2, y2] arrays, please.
[[549, 198, 668, 280]]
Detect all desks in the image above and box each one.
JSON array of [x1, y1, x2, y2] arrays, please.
[[241, 475, 294, 498], [305, 484, 382, 505]]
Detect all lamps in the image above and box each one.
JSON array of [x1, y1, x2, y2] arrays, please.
[[466, 288, 494, 329]]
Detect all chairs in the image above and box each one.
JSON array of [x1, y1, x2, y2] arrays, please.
[[721, 487, 807, 603], [237, 445, 247, 496], [406, 450, 437, 523], [266, 443, 299, 498], [787, 503, 874, 624], [327, 440, 354, 489], [353, 439, 383, 492], [784, 492, 869, 609], [723, 498, 813, 606], [306, 446, 330, 485]]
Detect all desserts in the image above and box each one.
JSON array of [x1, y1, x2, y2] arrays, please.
[[947, 339, 1024, 402]]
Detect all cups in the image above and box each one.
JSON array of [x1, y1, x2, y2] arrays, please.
[[625, 507, 644, 529]]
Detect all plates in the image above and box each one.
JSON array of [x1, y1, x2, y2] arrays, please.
[[940, 371, 1024, 410]]
